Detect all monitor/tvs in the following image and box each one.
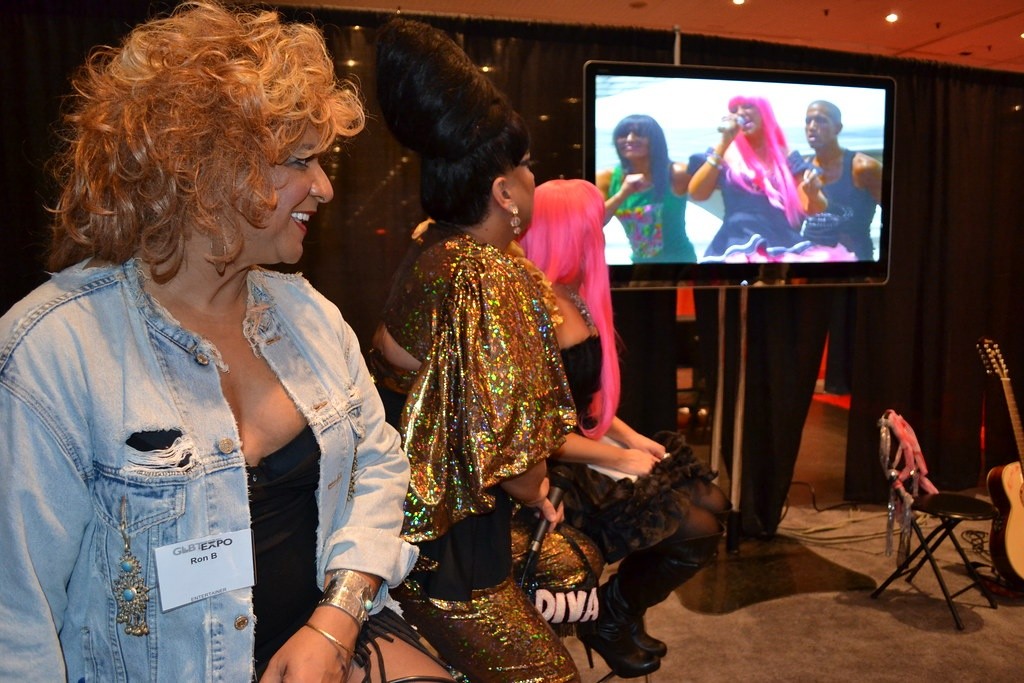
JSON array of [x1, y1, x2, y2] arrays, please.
[[582, 61, 896, 289]]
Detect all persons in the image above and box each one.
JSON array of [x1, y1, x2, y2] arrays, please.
[[363, 20, 732, 683], [591, 95, 883, 263], [0, 5, 459, 683]]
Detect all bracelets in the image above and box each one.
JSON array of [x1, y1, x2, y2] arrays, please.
[[706, 157, 723, 170], [306, 623, 357, 658], [710, 152, 724, 161], [318, 570, 374, 630]]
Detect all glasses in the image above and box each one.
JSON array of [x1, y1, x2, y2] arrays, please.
[[518, 154, 543, 168]]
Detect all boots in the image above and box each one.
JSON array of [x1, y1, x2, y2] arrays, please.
[[575, 500, 733, 678]]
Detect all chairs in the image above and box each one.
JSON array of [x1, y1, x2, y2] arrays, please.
[[870, 410, 999, 631]]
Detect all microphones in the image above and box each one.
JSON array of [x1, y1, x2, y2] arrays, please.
[[718, 117, 745, 133], [528, 466, 572, 561]]
